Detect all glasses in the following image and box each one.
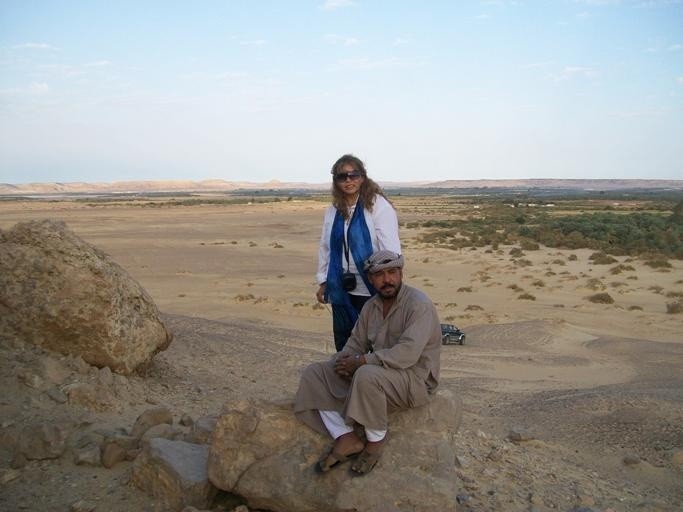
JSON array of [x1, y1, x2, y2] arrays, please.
[[333, 170, 360, 182]]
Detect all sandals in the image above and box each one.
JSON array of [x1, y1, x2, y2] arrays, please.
[[314, 449, 379, 477]]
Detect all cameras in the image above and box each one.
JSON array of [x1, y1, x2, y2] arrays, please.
[[342, 272, 356, 291]]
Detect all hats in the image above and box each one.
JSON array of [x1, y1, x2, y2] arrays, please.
[[363, 250, 404, 274]]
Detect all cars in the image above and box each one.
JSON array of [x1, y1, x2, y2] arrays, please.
[[440, 323, 466, 345]]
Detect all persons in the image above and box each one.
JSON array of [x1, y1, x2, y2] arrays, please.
[[294, 250, 441, 476], [317, 155, 401, 353]]
